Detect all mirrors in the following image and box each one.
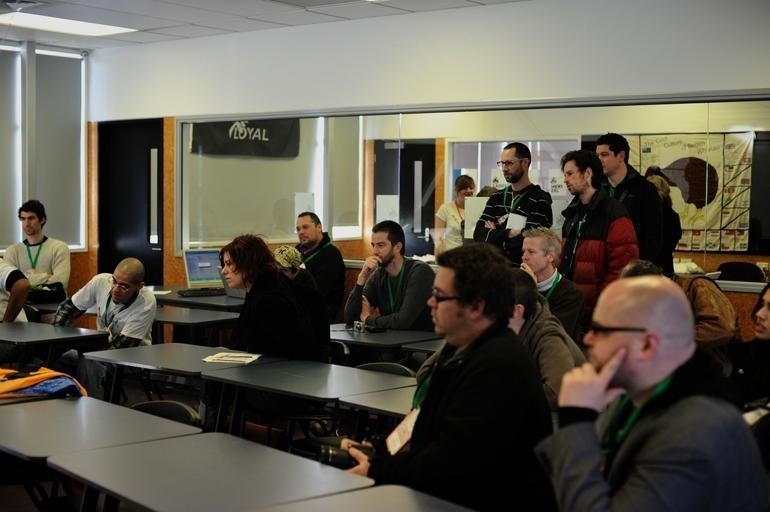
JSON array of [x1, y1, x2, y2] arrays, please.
[[172, 86, 770, 294]]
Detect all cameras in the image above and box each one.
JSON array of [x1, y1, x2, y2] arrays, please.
[[315, 444, 374, 469], [353, 321, 365, 332]]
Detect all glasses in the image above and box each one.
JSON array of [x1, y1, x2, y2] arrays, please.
[[430, 286, 459, 305], [586, 322, 645, 338], [496, 159, 522, 169], [106, 275, 129, 293]]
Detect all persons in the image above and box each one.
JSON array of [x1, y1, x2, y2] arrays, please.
[[52, 257, 157, 399], [1, 259, 30, 325], [197, 232, 331, 434], [270, 133, 770, 511], [1, 199, 72, 327]]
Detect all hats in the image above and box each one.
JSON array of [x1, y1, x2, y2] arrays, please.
[[272, 245, 301, 269]]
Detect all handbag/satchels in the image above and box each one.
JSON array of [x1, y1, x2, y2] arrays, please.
[[27, 281, 66, 303]]
